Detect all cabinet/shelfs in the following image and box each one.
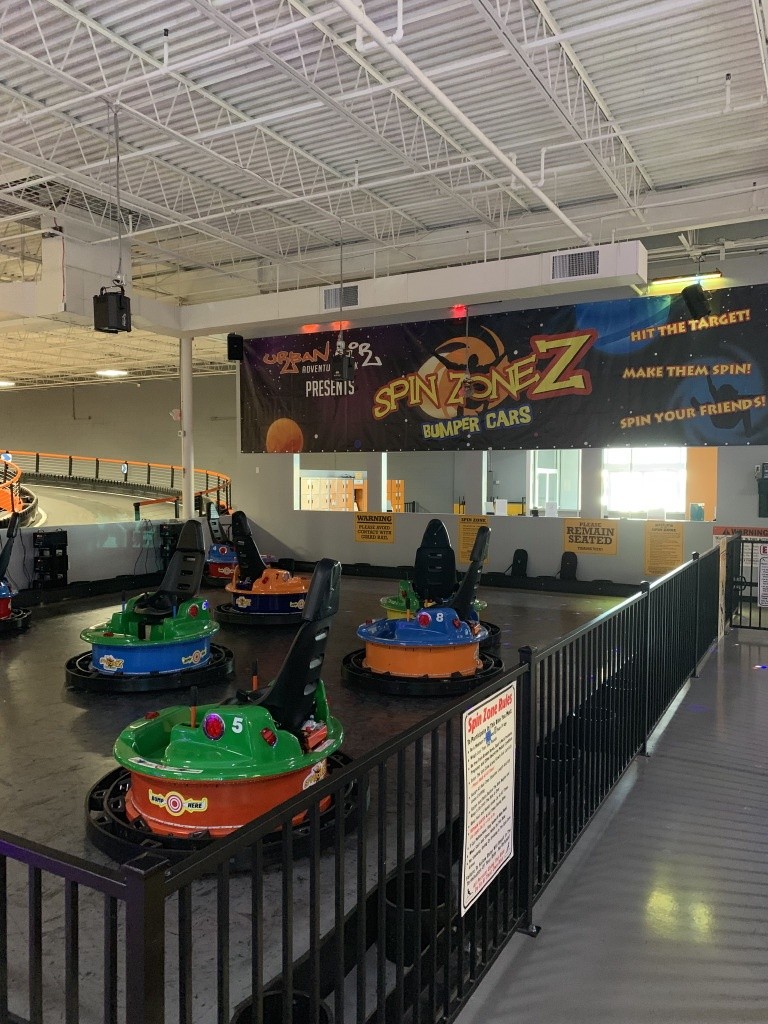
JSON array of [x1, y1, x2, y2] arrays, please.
[[299, 476, 406, 512]]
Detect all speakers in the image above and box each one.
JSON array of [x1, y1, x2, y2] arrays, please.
[[758, 478, 768, 518], [94, 285, 132, 334]]
